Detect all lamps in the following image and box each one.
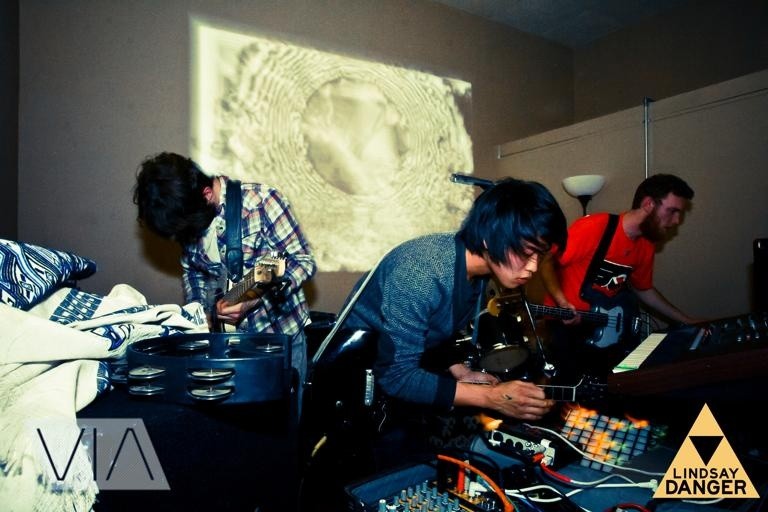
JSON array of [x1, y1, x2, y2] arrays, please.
[[561, 173, 607, 217]]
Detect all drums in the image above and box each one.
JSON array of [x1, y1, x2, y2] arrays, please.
[[472, 310, 529, 373]]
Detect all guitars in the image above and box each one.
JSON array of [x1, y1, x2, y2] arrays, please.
[[488, 289, 641, 352], [204, 251, 287, 334], [304, 327, 609, 438]]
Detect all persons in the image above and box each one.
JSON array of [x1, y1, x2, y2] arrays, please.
[[537, 173, 714, 349], [130, 148, 318, 423], [301, 174, 568, 512]]
